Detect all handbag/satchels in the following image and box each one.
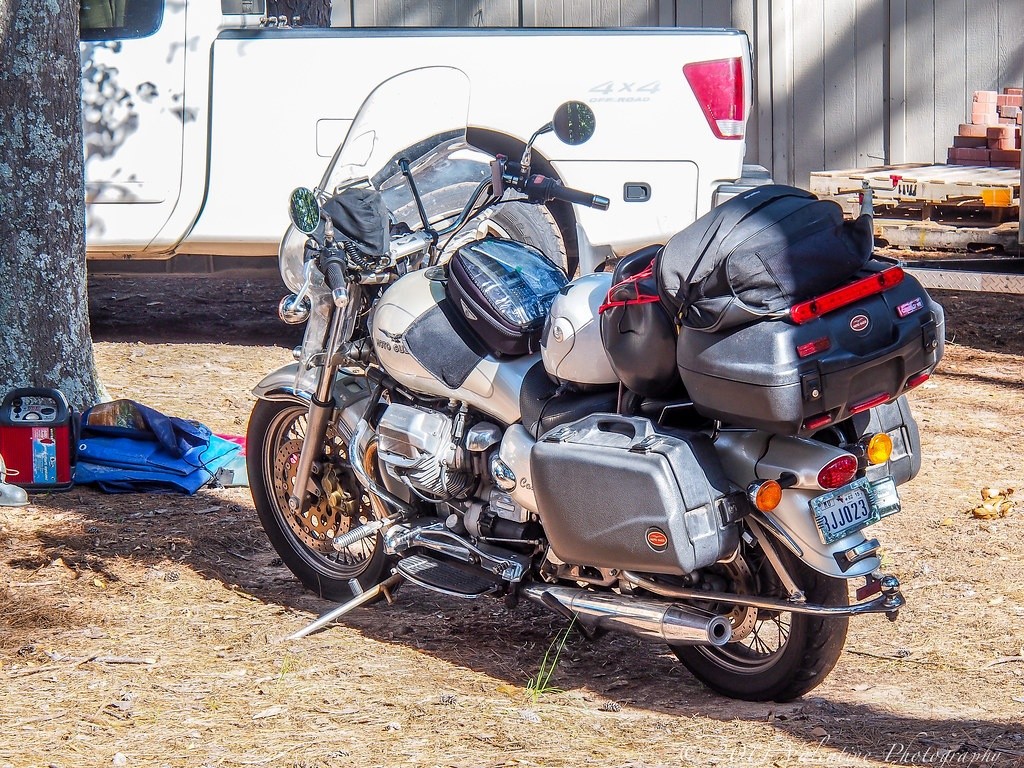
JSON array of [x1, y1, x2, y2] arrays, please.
[[652, 185, 876, 334], [597, 243, 689, 403], [443, 236, 571, 364], [73, 398, 213, 460]]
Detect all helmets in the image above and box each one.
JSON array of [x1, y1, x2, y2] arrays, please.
[[540, 271, 626, 396]]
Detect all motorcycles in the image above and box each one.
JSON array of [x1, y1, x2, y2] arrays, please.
[[246, 66, 947, 703]]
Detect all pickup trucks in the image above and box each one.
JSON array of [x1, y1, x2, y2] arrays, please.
[[78, 0, 775, 259]]
